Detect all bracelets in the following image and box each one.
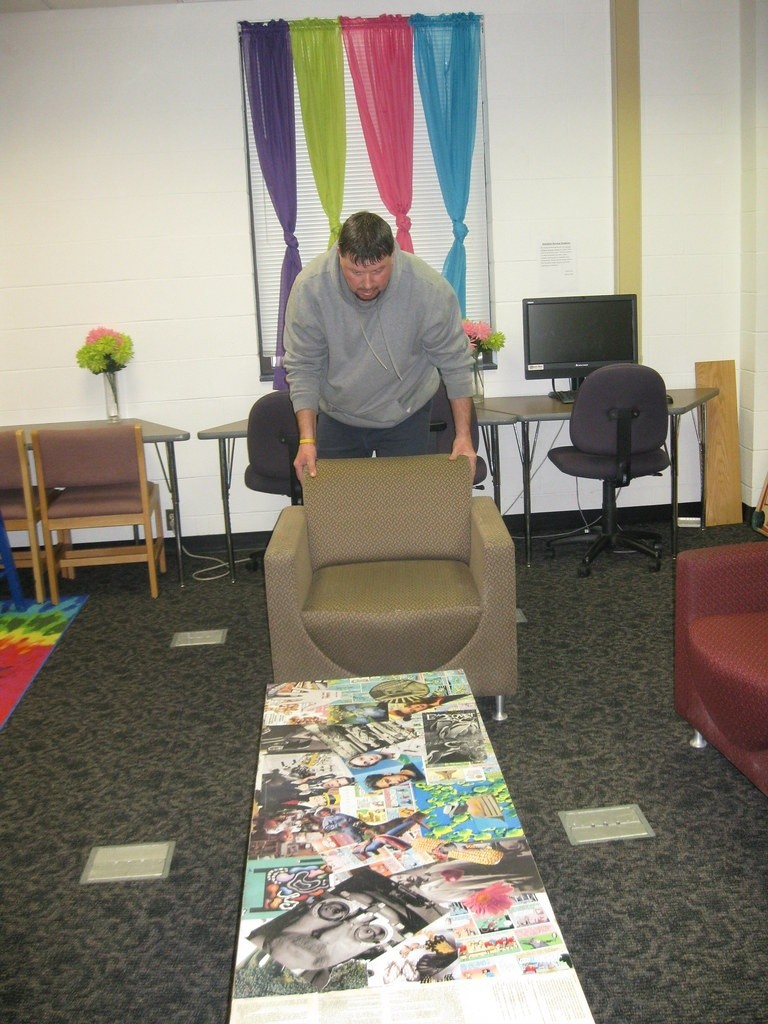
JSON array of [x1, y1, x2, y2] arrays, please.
[[299, 438, 317, 444]]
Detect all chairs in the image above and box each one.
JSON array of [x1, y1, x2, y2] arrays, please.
[[244, 391, 300, 496], [0, 423, 167, 605], [542, 363, 668, 576]]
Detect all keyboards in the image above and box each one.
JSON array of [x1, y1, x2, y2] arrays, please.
[[557, 390, 576, 404]]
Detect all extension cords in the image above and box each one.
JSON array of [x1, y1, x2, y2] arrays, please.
[[250, 549, 267, 565]]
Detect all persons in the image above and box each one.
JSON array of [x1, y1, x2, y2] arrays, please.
[[227, 680, 576, 1004], [282, 212, 481, 491]]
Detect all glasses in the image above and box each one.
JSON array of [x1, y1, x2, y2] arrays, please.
[[312, 897, 394, 946]]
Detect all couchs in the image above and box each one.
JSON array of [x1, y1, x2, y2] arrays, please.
[[676, 541, 768, 798], [264, 455, 516, 722]]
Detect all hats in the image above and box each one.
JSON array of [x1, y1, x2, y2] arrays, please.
[[323, 793, 340, 807], [364, 773, 384, 790], [314, 805, 333, 818]]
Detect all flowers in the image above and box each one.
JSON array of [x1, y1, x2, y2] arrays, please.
[[462, 319, 504, 351], [75, 324, 133, 374]]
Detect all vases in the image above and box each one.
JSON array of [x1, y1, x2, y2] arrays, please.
[[102, 371, 121, 421], [471, 353, 484, 403]]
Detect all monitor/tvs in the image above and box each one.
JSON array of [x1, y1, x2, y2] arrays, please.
[[522, 293, 637, 390]]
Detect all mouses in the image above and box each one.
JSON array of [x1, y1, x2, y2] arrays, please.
[[666, 394, 673, 403]]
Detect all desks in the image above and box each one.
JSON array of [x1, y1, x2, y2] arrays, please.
[[0, 418, 191, 588], [231, 669, 594, 1022], [198, 389, 719, 582]]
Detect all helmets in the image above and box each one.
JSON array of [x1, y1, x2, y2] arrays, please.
[[349, 863, 446, 929]]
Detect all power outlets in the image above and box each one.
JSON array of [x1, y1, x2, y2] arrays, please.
[[166, 508, 177, 532]]
